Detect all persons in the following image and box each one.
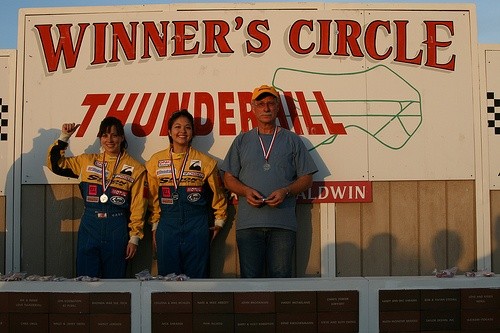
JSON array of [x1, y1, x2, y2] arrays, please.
[[219, 86, 318, 278], [145, 110, 228, 279], [45, 116, 147, 279]]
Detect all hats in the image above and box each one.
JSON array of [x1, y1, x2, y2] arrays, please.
[[252, 85, 279, 100]]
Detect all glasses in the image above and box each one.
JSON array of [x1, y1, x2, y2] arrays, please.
[[253, 102, 277, 106]]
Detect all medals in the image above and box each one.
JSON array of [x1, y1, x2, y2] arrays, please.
[[171, 192, 179, 200], [99, 194, 108, 203], [264, 162, 270, 170]]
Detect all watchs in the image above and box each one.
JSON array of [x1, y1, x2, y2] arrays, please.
[[285, 187, 291, 198]]
